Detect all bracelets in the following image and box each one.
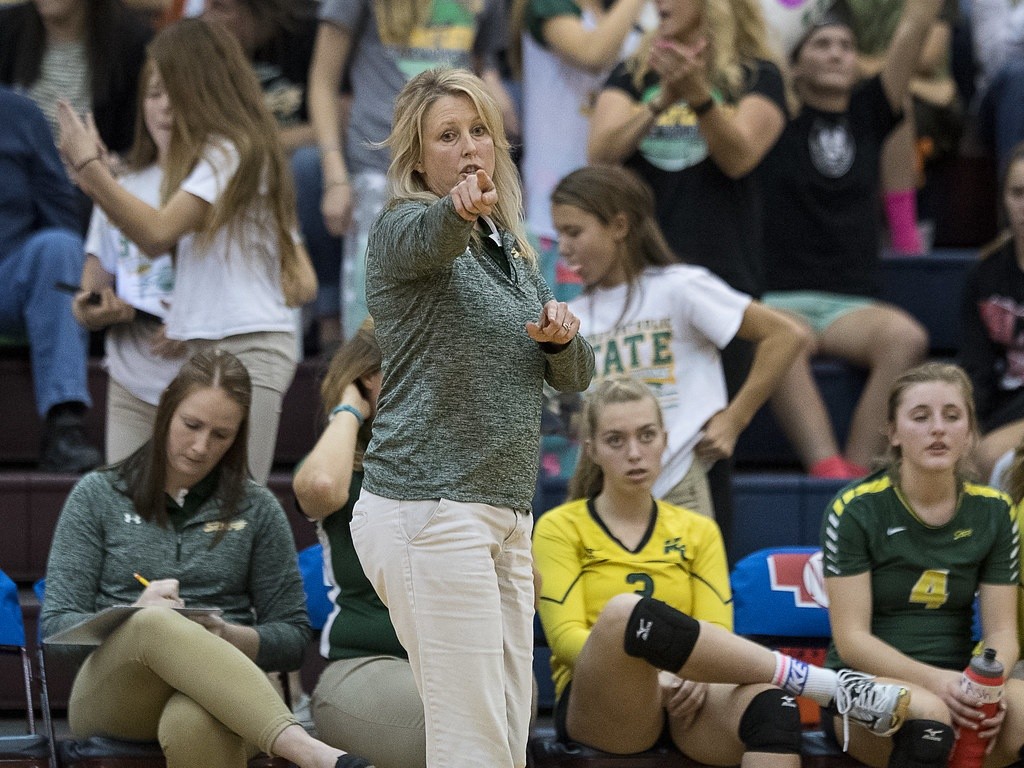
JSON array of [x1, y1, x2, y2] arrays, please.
[[327, 405, 365, 426], [689, 95, 717, 116], [73, 156, 100, 172], [649, 97, 667, 113]]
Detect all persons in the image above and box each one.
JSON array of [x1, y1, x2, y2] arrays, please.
[[0, 0, 1024, 768]]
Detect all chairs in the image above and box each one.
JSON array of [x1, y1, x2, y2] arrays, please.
[[0, 545, 848, 768]]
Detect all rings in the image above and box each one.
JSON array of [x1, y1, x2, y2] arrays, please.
[[562, 322, 571, 330]]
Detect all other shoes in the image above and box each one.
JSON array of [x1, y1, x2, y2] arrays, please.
[[43, 410, 99, 473], [335, 753, 376, 768], [808, 454, 868, 479]]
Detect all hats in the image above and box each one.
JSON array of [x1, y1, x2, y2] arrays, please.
[[787, 9, 845, 67]]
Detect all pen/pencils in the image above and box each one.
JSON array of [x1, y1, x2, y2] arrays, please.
[[132, 572, 185, 609]]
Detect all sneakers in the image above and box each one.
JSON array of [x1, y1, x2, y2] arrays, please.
[[828, 669, 911, 753]]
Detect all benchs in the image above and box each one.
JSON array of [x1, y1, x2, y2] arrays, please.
[[0, 356, 992, 714]]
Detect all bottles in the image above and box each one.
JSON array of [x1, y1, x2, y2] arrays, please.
[[944, 648, 1004, 768]]
